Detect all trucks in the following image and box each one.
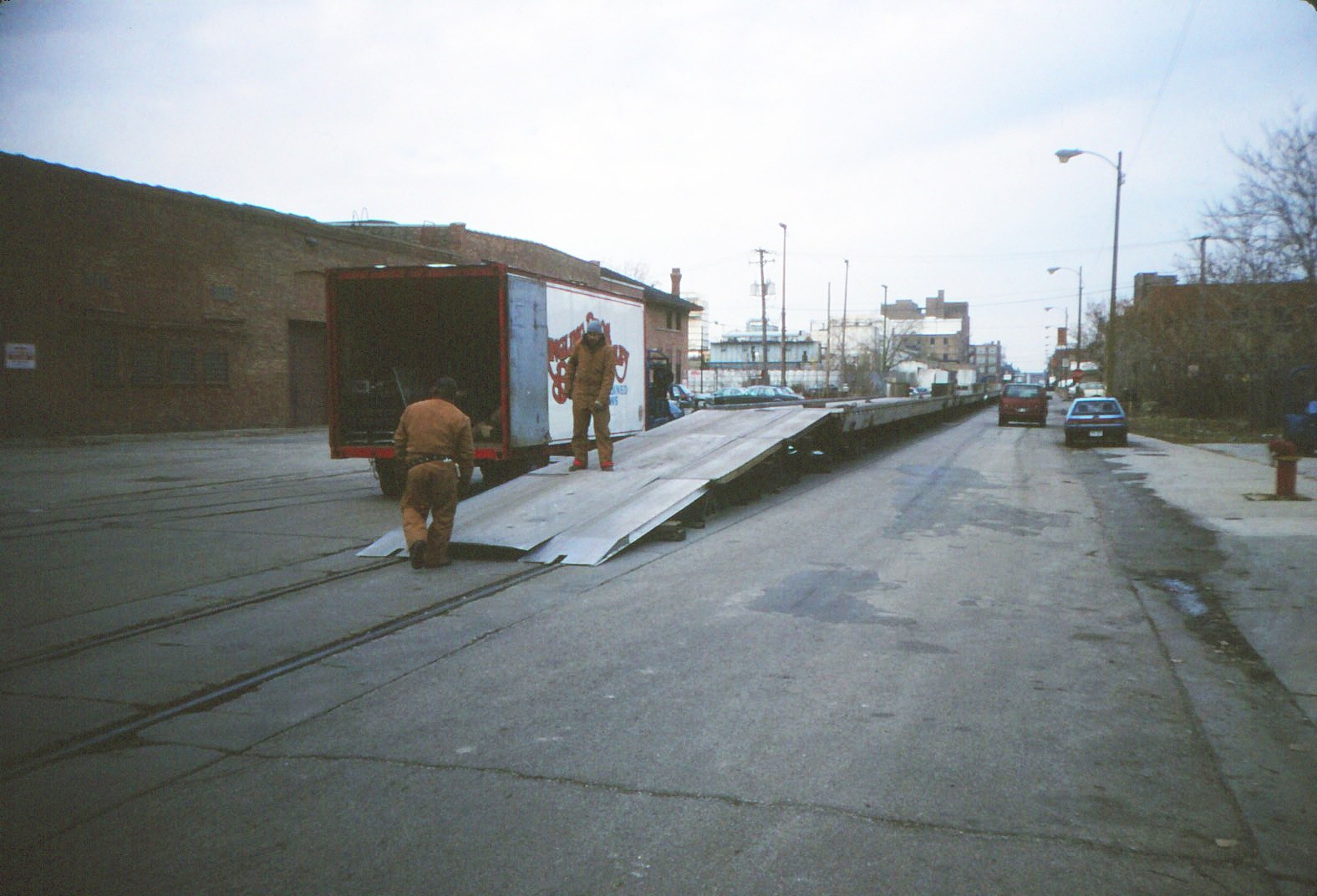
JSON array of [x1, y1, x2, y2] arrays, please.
[[322, 265, 684, 497]]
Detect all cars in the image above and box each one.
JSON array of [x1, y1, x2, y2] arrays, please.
[[673, 384, 806, 409], [909, 387, 927, 395], [998, 383, 1052, 427], [1061, 396, 1131, 447]]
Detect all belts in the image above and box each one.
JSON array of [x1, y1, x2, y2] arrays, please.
[[403, 455, 453, 470]]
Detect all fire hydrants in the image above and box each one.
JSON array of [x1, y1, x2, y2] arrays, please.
[[1268, 439, 1302, 497]]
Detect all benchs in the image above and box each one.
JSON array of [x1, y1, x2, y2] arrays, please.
[[1080, 404, 1114, 413]]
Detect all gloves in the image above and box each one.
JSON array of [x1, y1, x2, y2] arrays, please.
[[594, 400, 604, 413]]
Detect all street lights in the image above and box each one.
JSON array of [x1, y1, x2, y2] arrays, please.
[[1044, 306, 1068, 392], [1047, 265, 1082, 369], [880, 284, 887, 379], [1055, 148, 1124, 396], [778, 224, 788, 386], [841, 259, 849, 359]]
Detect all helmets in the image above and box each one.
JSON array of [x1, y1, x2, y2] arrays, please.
[[587, 320, 605, 334]]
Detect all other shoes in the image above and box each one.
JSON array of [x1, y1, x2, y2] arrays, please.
[[569, 466, 584, 472], [602, 466, 615, 472], [412, 540, 426, 570]]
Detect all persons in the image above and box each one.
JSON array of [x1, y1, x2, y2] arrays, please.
[[564, 321, 616, 471], [391, 376, 475, 570]]
